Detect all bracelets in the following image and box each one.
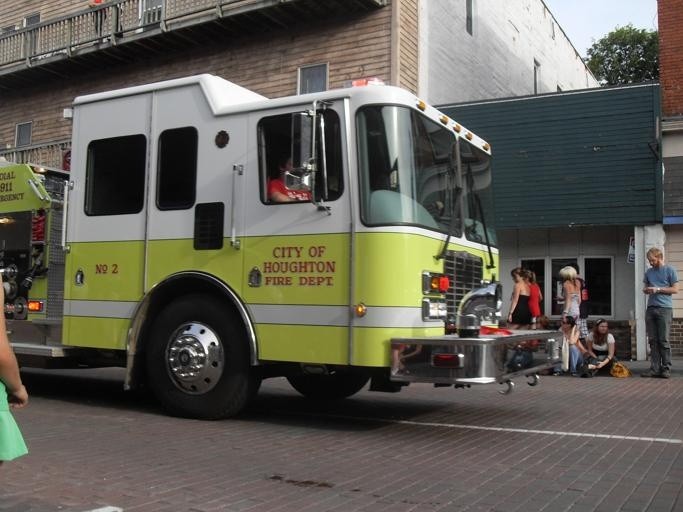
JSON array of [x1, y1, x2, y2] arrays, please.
[[657, 287, 661, 293]]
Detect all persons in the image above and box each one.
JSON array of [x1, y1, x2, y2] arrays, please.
[[551, 263, 618, 379], [639, 245, 681, 379], [522, 270, 542, 352], [0, 272, 29, 468], [267, 147, 311, 202], [504, 267, 534, 349]]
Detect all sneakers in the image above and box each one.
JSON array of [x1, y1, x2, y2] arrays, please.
[[640, 367, 673, 378]]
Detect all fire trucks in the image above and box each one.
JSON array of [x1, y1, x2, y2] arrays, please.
[[0, 73, 565, 421]]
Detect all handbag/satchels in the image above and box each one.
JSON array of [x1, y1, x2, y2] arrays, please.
[[609, 362, 633, 378]]
